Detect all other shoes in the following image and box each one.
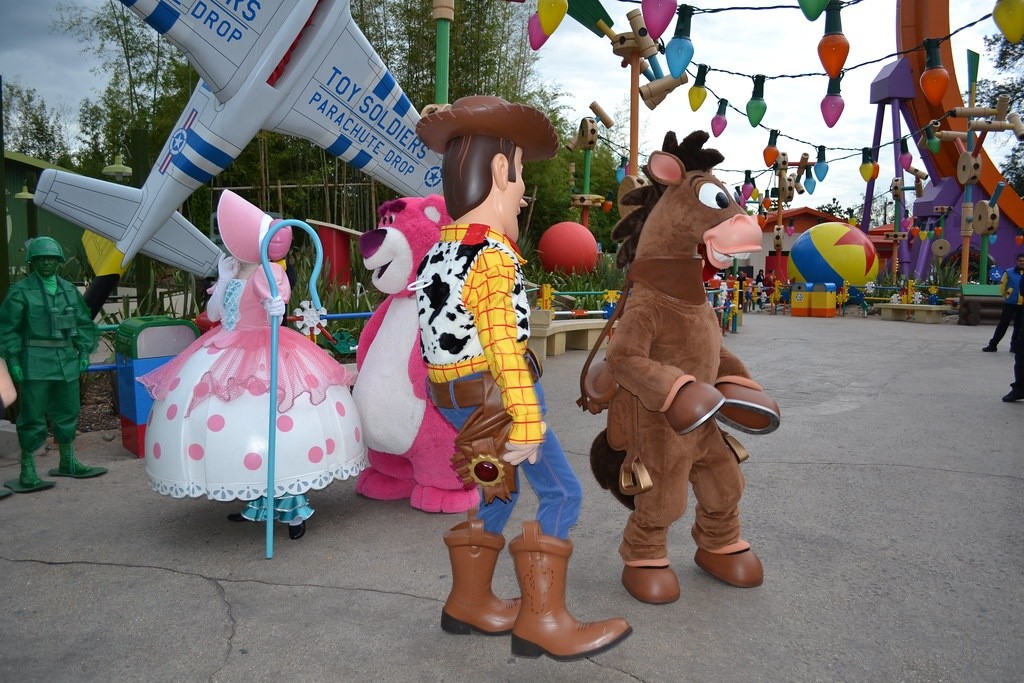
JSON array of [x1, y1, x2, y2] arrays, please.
[[983, 345, 997, 352], [1002, 383, 1024, 402], [289, 520, 305, 539], [227, 513, 249, 521]]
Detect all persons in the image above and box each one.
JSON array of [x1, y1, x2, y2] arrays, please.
[[1, 238, 92, 487], [756, 268, 776, 315], [738, 271, 748, 309], [1002, 275, 1024, 401], [414, 97, 633, 661], [982, 254, 1024, 353], [135, 190, 370, 539]]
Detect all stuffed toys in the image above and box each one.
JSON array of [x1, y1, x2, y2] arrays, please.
[[577, 130, 781, 603], [353, 194, 481, 514]]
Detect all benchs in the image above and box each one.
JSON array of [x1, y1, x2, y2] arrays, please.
[[527, 310, 620, 361], [873, 303, 951, 324]]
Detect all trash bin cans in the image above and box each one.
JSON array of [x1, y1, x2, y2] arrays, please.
[[194, 310, 221, 336], [791, 282, 813, 317], [114, 314, 201, 458], [811, 283, 837, 317]]
